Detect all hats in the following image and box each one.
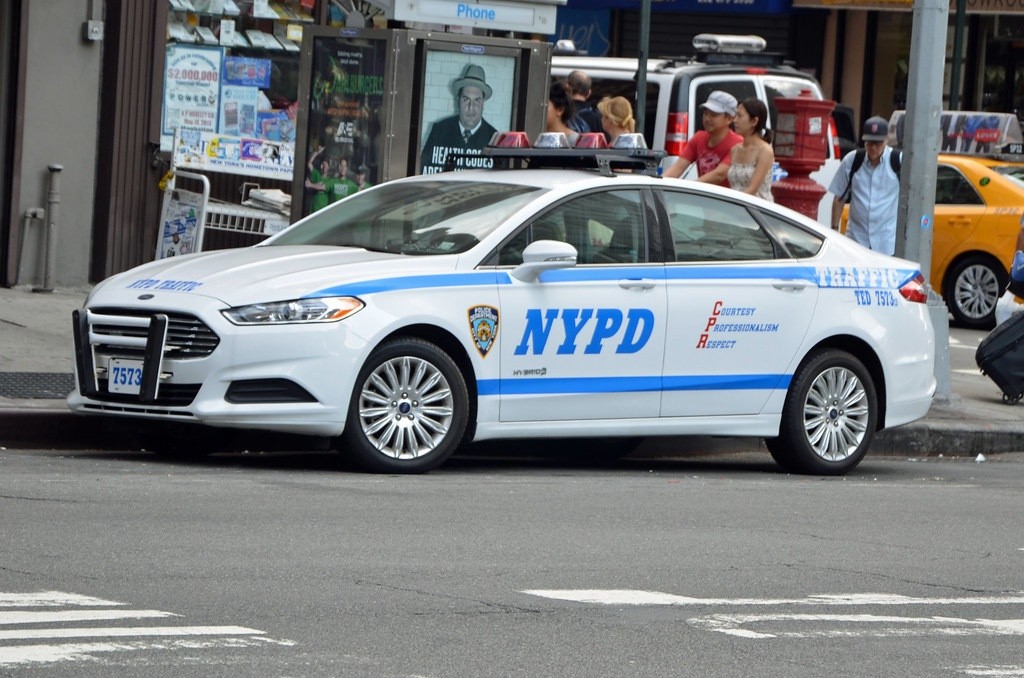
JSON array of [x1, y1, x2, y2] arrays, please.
[[597, 97, 632, 127], [701, 90, 738, 116], [862, 116, 889, 141], [451, 65, 493, 101]]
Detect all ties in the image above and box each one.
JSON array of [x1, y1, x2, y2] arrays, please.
[[464, 130, 470, 143]]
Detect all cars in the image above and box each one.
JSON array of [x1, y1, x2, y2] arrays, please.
[[65, 131, 937, 477], [841, 111, 1024, 331]]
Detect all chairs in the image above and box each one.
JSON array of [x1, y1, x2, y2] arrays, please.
[[531, 222, 563, 242], [594, 217, 634, 264], [692, 213, 747, 261]]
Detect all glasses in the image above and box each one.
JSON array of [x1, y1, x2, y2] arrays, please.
[[460, 95, 482, 104]]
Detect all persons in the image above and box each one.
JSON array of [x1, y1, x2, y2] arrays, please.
[[545, 71, 636, 175], [828, 115, 903, 255], [661, 90, 774, 202], [304, 146, 372, 214], [420, 65, 499, 177]]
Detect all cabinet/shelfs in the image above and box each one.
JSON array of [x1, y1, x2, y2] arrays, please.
[[154, 167, 292, 260]]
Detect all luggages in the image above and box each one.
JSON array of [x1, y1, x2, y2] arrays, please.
[[976, 310, 1024, 403]]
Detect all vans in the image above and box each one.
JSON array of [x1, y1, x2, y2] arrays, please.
[[549, 35, 842, 235]]
